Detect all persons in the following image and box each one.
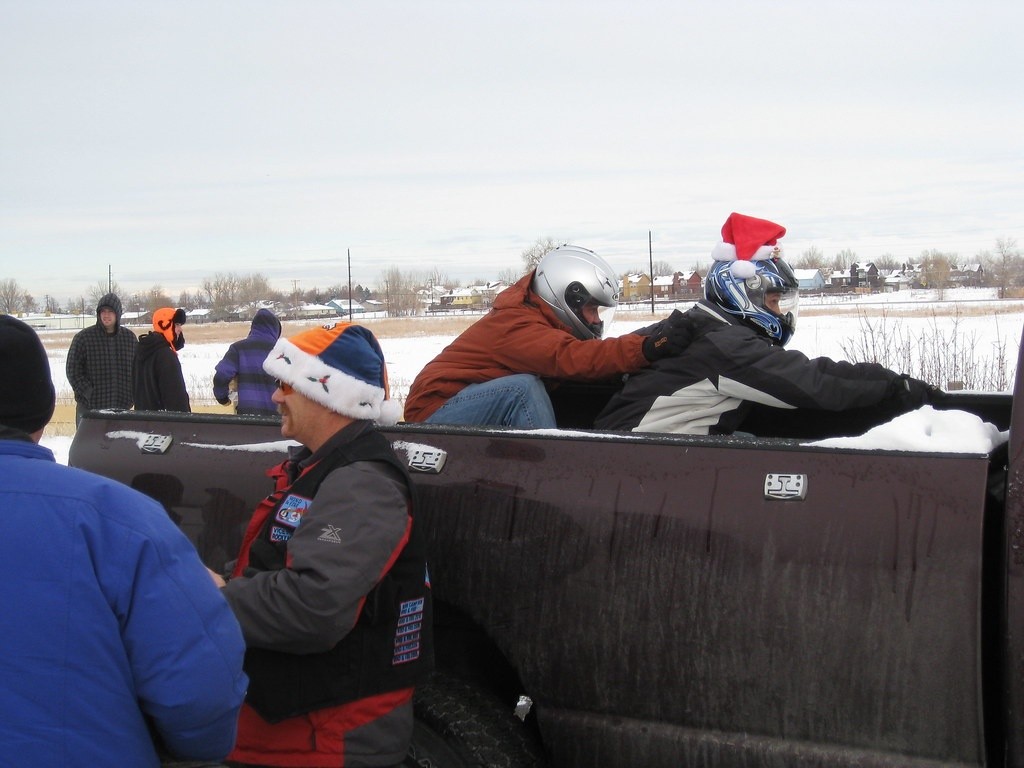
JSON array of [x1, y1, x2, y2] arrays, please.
[[593, 212, 957, 444], [402, 243, 698, 428], [132, 306, 192, 413], [197, 323, 430, 768], [66, 292, 136, 429], [0, 314, 249, 768], [211, 308, 284, 417]]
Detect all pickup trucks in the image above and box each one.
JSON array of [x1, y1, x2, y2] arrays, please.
[[68, 320, 1024, 768]]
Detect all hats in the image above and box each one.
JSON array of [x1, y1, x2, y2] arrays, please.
[[0, 314, 55, 434], [152, 308, 186, 356], [262, 322, 403, 426], [711, 212, 786, 279]]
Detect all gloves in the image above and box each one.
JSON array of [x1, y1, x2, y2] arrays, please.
[[643, 318, 694, 362], [884, 373, 946, 412]]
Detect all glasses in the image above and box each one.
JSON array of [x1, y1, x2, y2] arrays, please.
[[274, 379, 285, 391]]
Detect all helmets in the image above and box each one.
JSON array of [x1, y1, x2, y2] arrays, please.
[[704, 256, 799, 347], [530, 245, 619, 341]]
